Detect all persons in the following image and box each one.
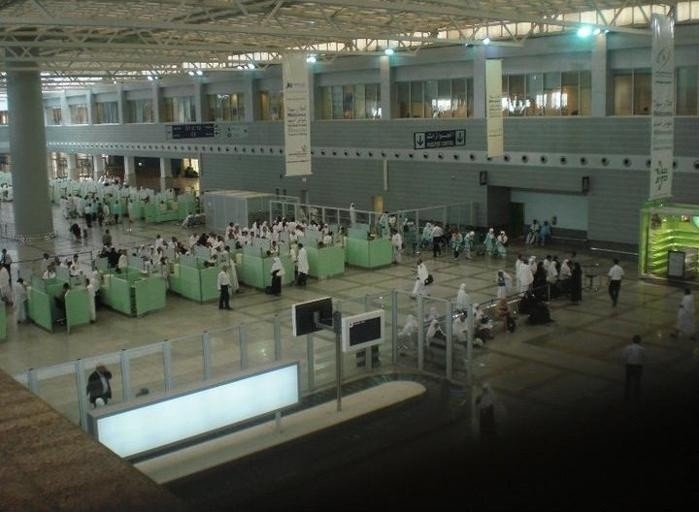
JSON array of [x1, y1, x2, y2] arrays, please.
[[380, 211, 408, 233], [87, 363, 114, 408], [515, 253, 582, 305], [1, 246, 129, 324], [669, 287, 698, 342], [423, 221, 508, 259], [527, 220, 551, 246], [62, 182, 132, 248], [495, 270, 515, 333], [152, 235, 233, 310], [226, 223, 284, 294], [621, 334, 646, 403], [475, 383, 496, 440], [409, 260, 430, 299], [391, 227, 401, 264], [251, 215, 333, 286], [605, 259, 625, 307], [400, 284, 494, 347]]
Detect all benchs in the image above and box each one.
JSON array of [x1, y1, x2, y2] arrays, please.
[[526, 225, 589, 249], [397, 300, 550, 372]]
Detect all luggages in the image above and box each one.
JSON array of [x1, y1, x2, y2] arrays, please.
[[476, 243, 487, 255]]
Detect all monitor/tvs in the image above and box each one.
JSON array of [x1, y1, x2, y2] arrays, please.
[[342, 309, 385, 352], [292, 295, 333, 337]]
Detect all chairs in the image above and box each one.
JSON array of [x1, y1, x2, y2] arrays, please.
[[52, 296, 66, 325]]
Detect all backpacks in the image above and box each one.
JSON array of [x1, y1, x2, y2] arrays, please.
[[507, 314, 516, 335]]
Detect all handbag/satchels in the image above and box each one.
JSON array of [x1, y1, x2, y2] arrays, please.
[[454, 251, 459, 259], [502, 242, 509, 247], [424, 273, 434, 286], [498, 278, 506, 287]]
